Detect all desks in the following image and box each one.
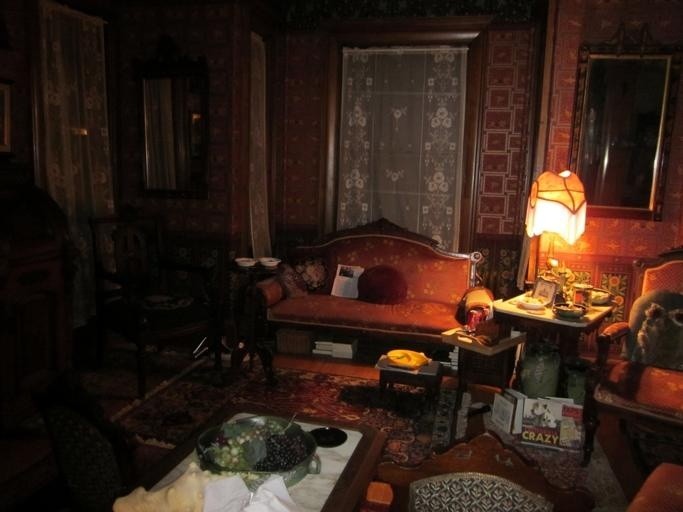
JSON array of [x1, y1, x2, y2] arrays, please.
[[230, 262, 280, 368], [126, 407, 389, 512]]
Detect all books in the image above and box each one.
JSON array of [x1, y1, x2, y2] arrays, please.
[[311, 336, 359, 360], [448, 346, 459, 371], [490, 388, 583, 455]]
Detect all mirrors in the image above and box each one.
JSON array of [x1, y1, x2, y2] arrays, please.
[[131, 27, 209, 201], [566, 22, 683, 222]]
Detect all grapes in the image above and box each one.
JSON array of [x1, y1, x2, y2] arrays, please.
[[254, 433, 308, 471]]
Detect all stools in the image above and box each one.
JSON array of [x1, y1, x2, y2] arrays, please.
[[455, 344, 517, 408], [379, 359, 443, 412]]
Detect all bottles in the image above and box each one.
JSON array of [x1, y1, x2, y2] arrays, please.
[[518, 344, 594, 405]]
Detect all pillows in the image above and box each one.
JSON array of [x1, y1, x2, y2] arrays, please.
[[272, 255, 408, 304], [620, 290, 683, 370], [128, 432, 176, 474]]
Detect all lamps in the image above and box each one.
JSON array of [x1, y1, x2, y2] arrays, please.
[[525, 169, 587, 277]]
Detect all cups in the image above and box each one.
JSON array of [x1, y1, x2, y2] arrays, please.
[[573, 282, 592, 309]]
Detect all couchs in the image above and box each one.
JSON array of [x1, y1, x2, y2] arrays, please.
[[243, 217, 495, 361]]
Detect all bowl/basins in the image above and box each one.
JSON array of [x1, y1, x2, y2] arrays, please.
[[195, 416, 321, 491], [517, 296, 586, 320], [585, 287, 614, 306], [234, 257, 280, 268]]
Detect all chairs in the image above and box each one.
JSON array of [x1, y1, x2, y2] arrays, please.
[[29, 372, 194, 512], [582, 247, 683, 468], [360, 430, 595, 512], [87, 214, 225, 400]]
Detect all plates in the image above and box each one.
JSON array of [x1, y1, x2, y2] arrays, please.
[[305, 426, 348, 449], [385, 350, 426, 367]]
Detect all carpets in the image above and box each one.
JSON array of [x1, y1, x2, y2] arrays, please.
[[0, 347, 683, 512]]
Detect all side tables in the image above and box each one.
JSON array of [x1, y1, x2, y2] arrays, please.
[[494, 289, 616, 398]]
